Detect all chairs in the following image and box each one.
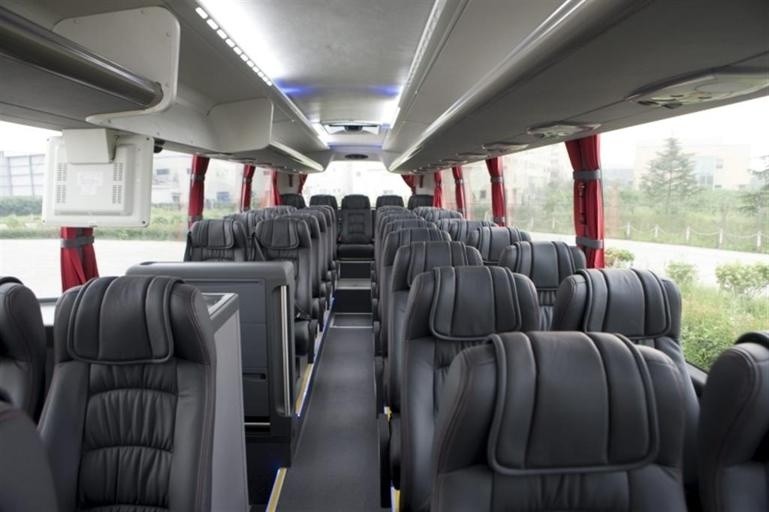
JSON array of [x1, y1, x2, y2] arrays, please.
[[1, 275, 52, 510], [338, 195, 374, 245], [37, 268, 216, 512], [2, 399, 57, 509], [184, 192, 338, 378]]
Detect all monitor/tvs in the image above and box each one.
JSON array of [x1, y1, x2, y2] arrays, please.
[[42, 128, 155, 228]]
